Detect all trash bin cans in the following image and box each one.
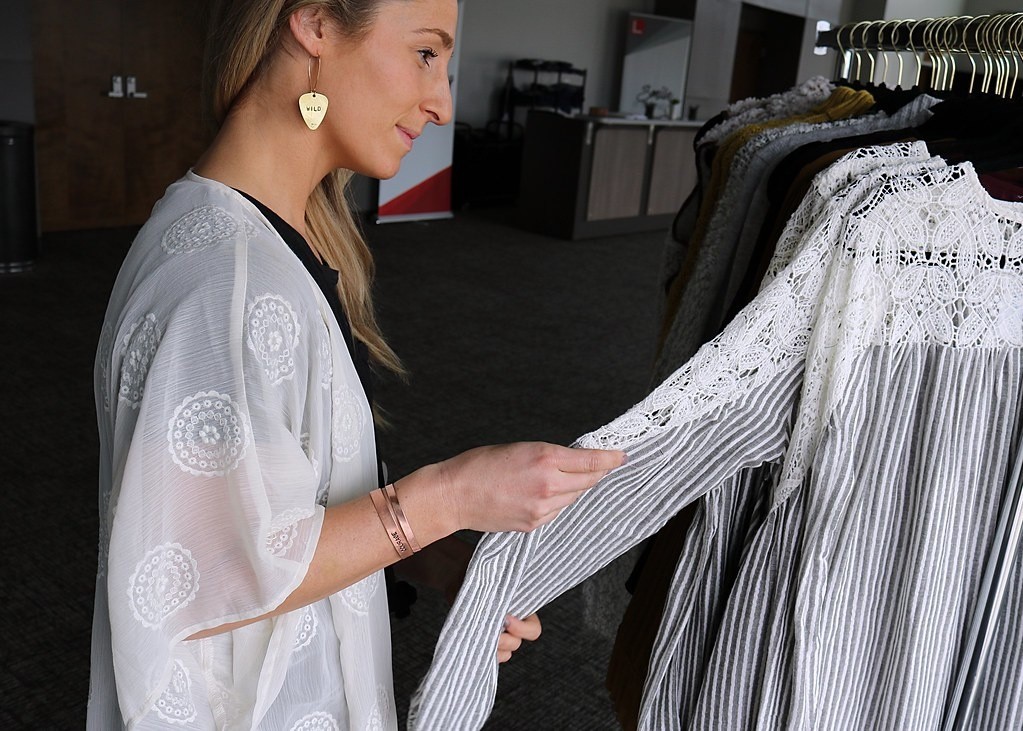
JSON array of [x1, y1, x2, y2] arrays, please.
[[0, 117, 44, 277]]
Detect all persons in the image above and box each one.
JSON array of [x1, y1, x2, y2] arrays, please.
[[87, 0, 627, 731]]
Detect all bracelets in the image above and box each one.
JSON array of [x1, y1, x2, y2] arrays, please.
[[368, 485, 421, 561]]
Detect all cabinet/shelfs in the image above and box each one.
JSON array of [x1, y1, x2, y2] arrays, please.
[[29, 1, 214, 237]]
